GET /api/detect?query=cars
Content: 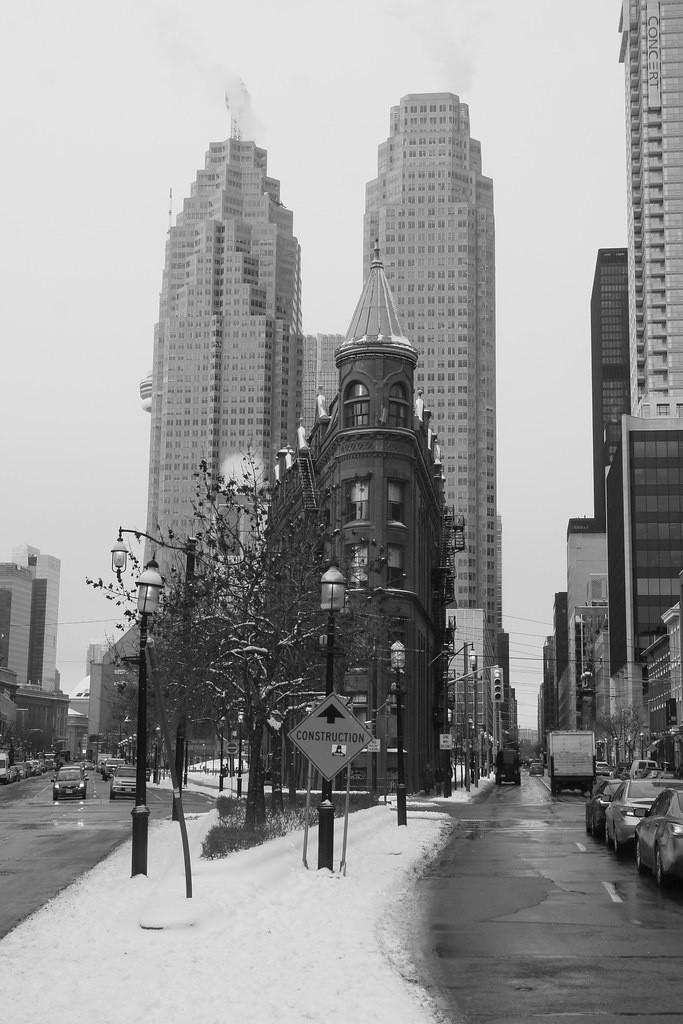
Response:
[51,761,89,800]
[604,779,683,855]
[585,779,632,839]
[110,764,148,800]
[97,757,127,781]
[635,787,683,889]
[82,761,95,771]
[595,759,683,779]
[10,759,48,782]
[529,763,544,776]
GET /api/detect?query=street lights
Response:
[468,716,500,784]
[131,553,164,872]
[111,526,198,821]
[389,637,407,826]
[316,553,347,870]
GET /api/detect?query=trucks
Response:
[496,749,520,785]
[44,753,57,770]
[546,729,595,796]
[0,753,11,784]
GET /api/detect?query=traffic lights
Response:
[490,668,504,702]
